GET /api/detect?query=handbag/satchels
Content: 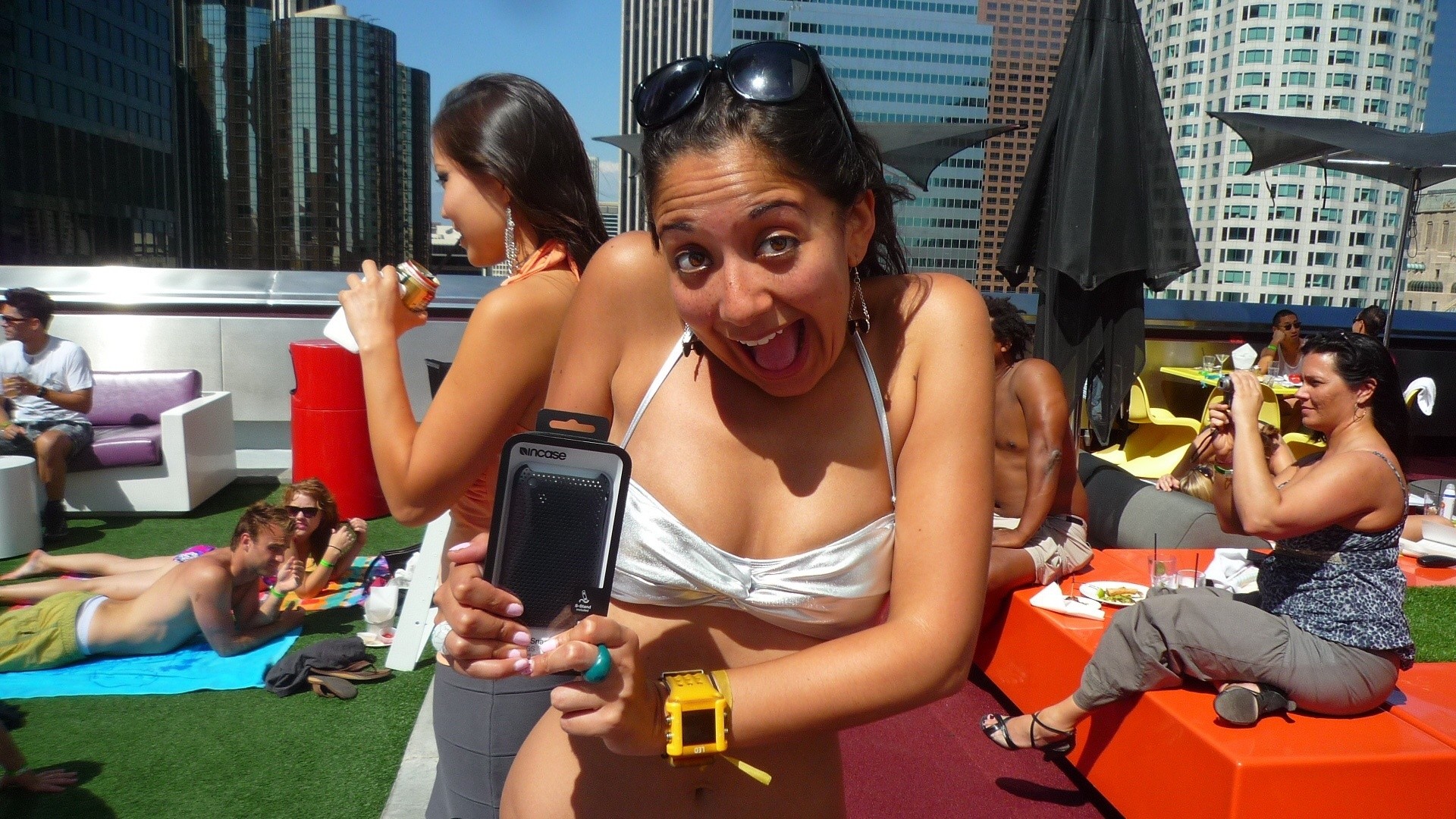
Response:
[364,541,423,583]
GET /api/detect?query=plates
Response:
[1079,581,1149,606]
[364,641,392,647]
[1063,595,1102,610]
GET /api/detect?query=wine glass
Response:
[1215,354,1229,377]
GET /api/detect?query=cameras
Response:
[1217,375,1234,408]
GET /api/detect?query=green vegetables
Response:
[1098,589,1136,603]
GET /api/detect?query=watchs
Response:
[658,669,772,786]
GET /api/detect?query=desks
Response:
[0,456,43,559]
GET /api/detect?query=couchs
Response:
[62,369,236,512]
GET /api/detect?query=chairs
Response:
[1081,366,1200,479]
[1201,371,1323,478]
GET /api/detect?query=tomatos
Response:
[1288,374,1302,384]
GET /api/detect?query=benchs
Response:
[999,544,1456,819]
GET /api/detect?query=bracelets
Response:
[36,386,47,398]
[319,559,336,567]
[1267,345,1277,351]
[1213,464,1233,474]
[270,587,287,598]
[0,421,13,430]
[327,545,342,553]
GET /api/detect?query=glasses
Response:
[1352,317,1362,324]
[1277,321,1302,330]
[1325,328,1357,358]
[284,505,319,519]
[0,313,30,326]
[633,39,852,163]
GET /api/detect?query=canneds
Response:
[395,258,440,314]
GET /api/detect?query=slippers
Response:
[310,658,391,680]
[307,676,358,700]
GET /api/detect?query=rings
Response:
[431,620,453,656]
[580,643,612,684]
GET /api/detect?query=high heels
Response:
[980,712,1076,761]
[1213,681,1298,725]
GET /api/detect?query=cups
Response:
[2,372,19,398]
[1253,366,1261,378]
[1203,356,1215,375]
[1148,554,1178,589]
[1424,493,1442,516]
[1262,374,1275,388]
[1057,573,1074,599]
[1177,569,1206,588]
[1268,361,1279,378]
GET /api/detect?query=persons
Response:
[336,71,610,819]
[1352,305,1397,364]
[429,42,996,819]
[0,477,368,673]
[1257,309,1309,375]
[979,328,1417,763]
[981,296,1094,635]
[1156,419,1298,504]
[0,287,97,536]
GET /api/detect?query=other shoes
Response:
[42,500,68,536]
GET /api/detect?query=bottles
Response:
[1439,483,1456,520]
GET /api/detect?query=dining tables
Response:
[1160,367,1302,395]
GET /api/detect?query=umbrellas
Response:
[1203,111,1456,351]
[591,123,1030,194]
[996,0,1202,473]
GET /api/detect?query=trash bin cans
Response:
[291,337,393,524]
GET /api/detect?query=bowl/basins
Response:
[357,632,377,644]
[1288,374,1302,383]
[379,627,397,643]
[1235,368,1251,372]
[366,614,394,637]
